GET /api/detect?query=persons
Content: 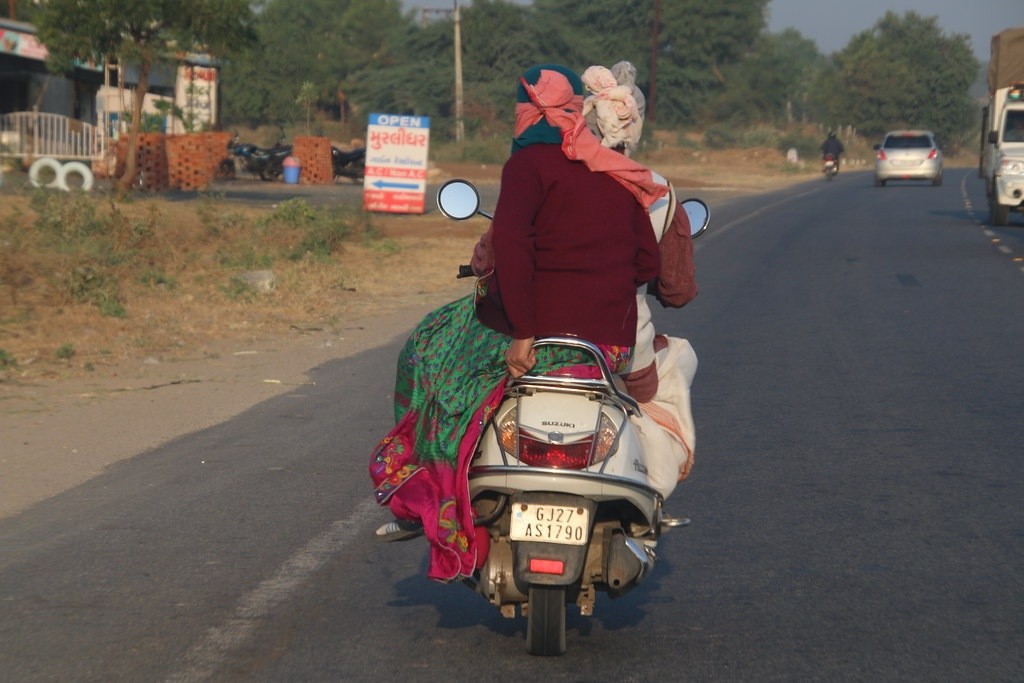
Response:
[369,64,659,587]
[473,62,697,496]
[822,131,844,170]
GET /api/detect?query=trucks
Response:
[978,82,1024,226]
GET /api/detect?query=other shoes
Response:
[375,520,418,541]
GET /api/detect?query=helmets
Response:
[829,131,836,138]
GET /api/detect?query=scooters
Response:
[822,153,839,182]
[437,178,711,656]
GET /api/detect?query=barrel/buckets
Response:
[283,165,301,185]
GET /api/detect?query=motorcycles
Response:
[214,130,293,182]
[331,146,365,181]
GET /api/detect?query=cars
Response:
[874,130,943,187]
